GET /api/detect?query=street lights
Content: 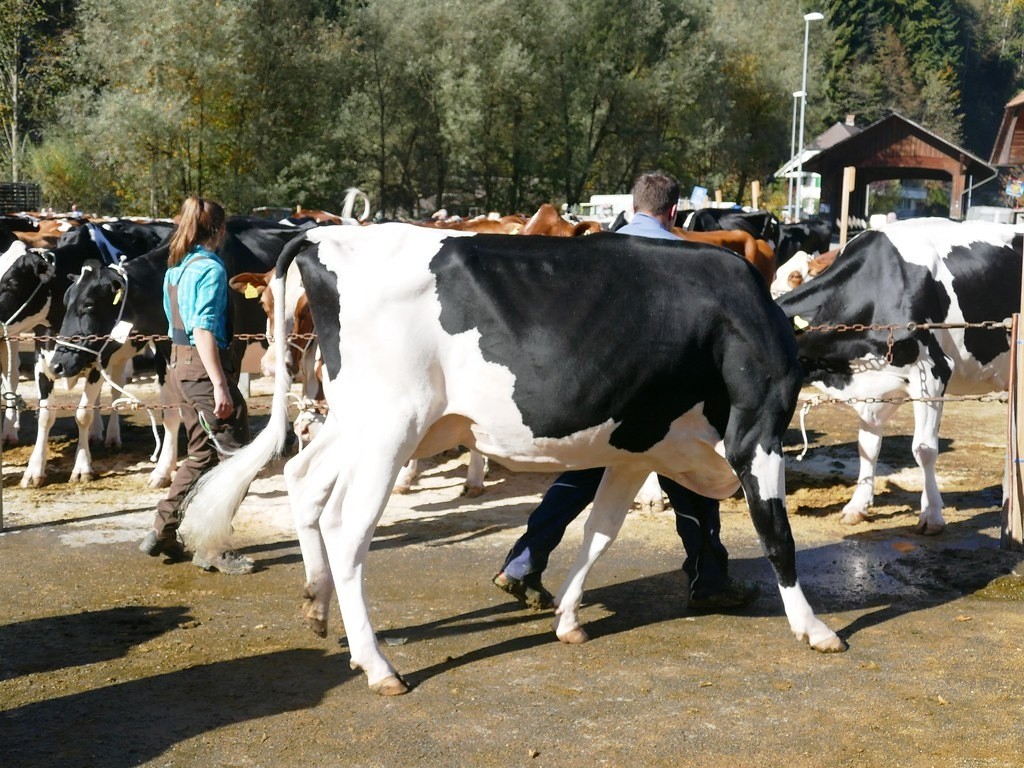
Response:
[785,91,807,225]
[794,12,826,221]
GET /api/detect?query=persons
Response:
[491,171,762,610]
[137,200,256,576]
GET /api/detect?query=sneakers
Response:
[493,570,552,608]
[193,547,255,575]
[689,577,760,609]
[139,530,184,558]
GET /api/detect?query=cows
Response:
[774,215,1023,535]
[0,184,862,489]
[178,220,849,697]
